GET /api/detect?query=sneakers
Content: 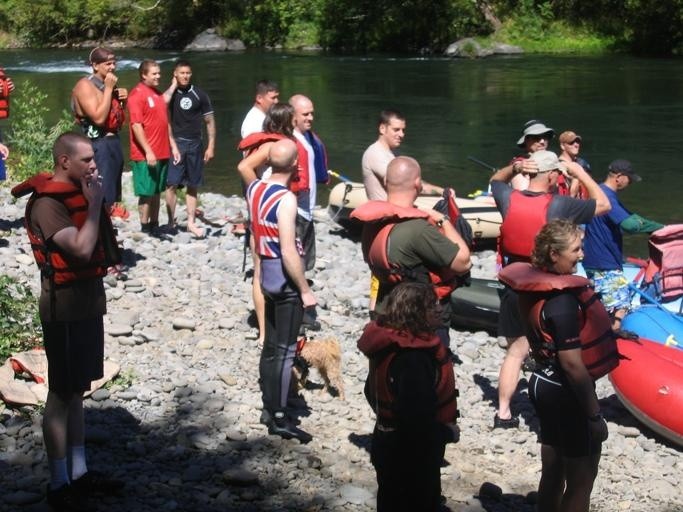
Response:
[260,410,301,427]
[268,421,313,443]
[495,415,519,428]
[302,314,321,331]
[46,483,98,510]
[76,474,124,496]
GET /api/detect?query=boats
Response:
[325,178,503,244]
[608,300,682,445]
[570,254,682,314]
[449,273,511,334]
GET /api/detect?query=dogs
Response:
[292,334,345,402]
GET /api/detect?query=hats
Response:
[517,118,555,144]
[559,131,583,146]
[608,158,642,183]
[84,46,115,64]
[530,151,563,172]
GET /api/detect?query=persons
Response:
[11,132,128,510]
[556,125,594,201]
[359,108,457,313]
[355,283,462,511]
[500,117,570,197]
[70,43,128,279]
[0,135,11,186]
[498,220,620,512]
[0,66,15,120]
[286,91,328,282]
[127,60,182,237]
[347,154,473,358]
[245,137,314,442]
[160,64,216,239]
[490,149,611,427]
[241,82,278,140]
[238,101,321,331]
[580,159,667,331]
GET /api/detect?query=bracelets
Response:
[586,412,604,423]
[512,164,521,174]
[436,215,452,228]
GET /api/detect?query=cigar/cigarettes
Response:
[90,174,104,179]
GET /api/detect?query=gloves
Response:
[445,422,460,444]
[577,410,608,441]
